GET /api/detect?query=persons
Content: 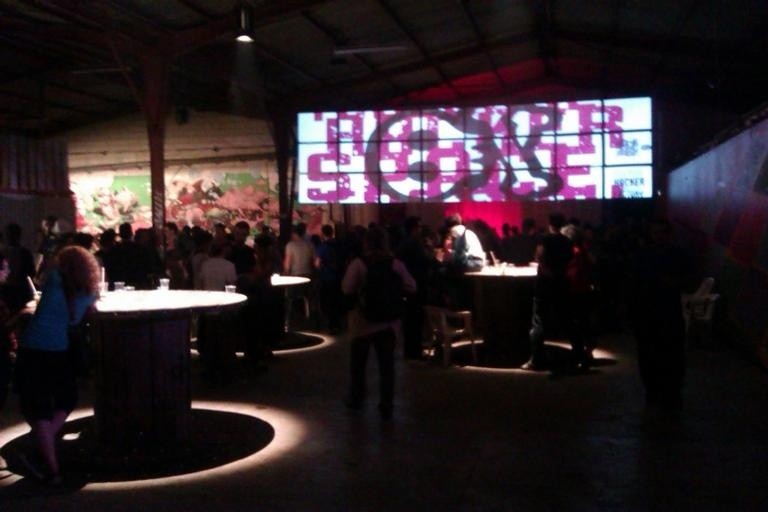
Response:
[0,208,723,428]
[11,243,109,495]
[624,217,720,444]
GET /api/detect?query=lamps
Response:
[232,1,257,44]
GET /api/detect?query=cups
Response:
[224,285,236,297]
[159,277,170,293]
[113,281,126,292]
[126,287,136,293]
[97,282,110,299]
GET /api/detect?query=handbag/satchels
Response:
[66,318,108,372]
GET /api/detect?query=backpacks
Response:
[355,255,405,322]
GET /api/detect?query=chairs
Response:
[423,303,479,366]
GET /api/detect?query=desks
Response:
[453,262,539,368]
[88,288,249,431]
[269,275,312,347]
